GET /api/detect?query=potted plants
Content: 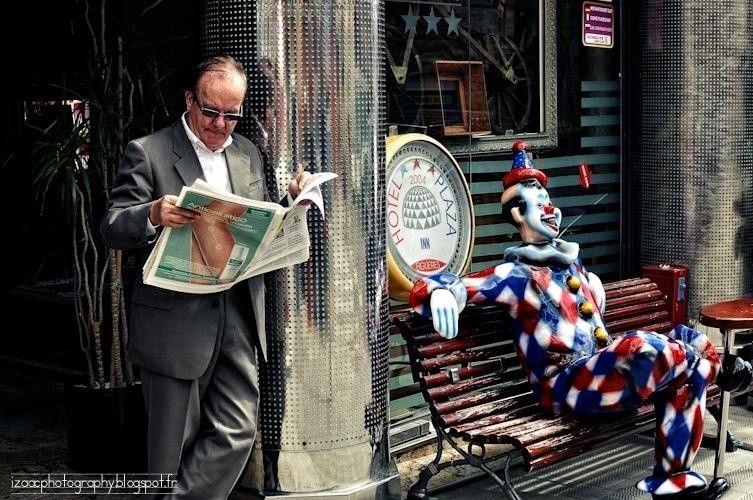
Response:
[20,0,172,473]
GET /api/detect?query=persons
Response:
[99,58,319,499]
[410,138,753,500]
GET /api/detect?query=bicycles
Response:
[384,5,532,134]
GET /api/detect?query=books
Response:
[142,171,338,296]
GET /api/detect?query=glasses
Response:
[193,94,244,122]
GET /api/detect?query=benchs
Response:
[387,264,738,500]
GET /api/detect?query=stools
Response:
[698,298,753,494]
